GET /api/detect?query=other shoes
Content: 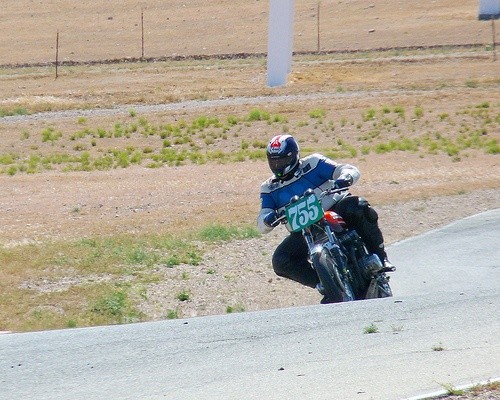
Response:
[316,283,326,295]
[383,259,396,271]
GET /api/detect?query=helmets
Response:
[266,134,299,177]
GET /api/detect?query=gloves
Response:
[264,210,280,227]
[334,173,353,195]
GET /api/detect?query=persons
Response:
[256,133,398,303]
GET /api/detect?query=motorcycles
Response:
[270,180,396,303]
